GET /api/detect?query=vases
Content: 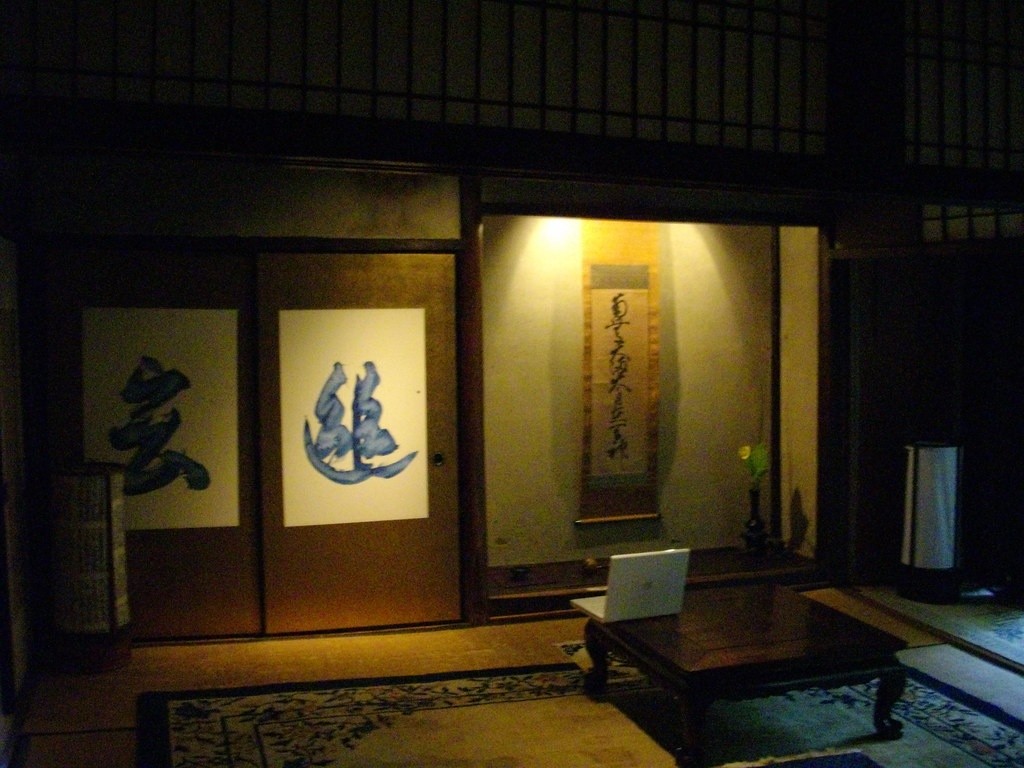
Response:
[740,488,768,551]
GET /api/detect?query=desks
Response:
[584,583,910,768]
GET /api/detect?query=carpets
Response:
[552,639,648,691]
[136,661,1024,768]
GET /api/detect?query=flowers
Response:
[739,443,770,489]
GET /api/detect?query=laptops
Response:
[570,548,690,624]
